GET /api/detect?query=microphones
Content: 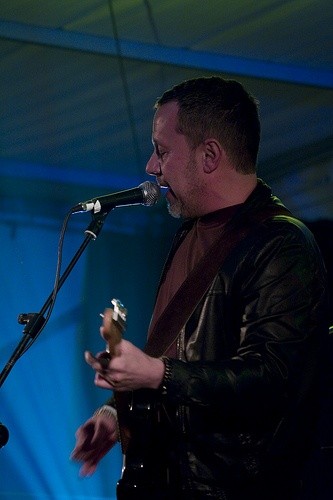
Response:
[71,181,162,214]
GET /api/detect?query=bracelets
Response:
[99,404,122,446]
[158,356,173,396]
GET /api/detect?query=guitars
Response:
[98,299,176,500]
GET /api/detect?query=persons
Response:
[73,77,333,500]
